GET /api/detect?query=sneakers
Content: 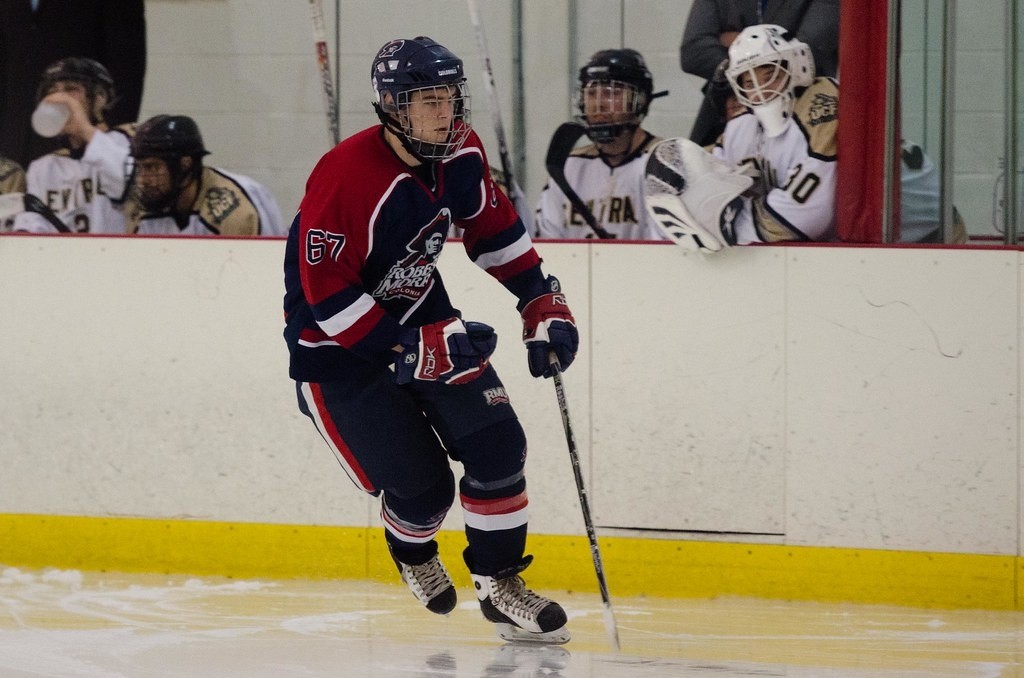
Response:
[385,529,458,617]
[462,546,570,645]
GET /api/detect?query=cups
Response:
[31,96,71,137]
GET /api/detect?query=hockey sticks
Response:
[544,121,618,243]
[548,350,620,650]
[0,191,71,233]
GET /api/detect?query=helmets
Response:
[725,23,816,139]
[571,48,671,143]
[123,114,210,209]
[369,37,472,164]
[37,57,115,107]
[709,59,736,111]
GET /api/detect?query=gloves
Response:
[516,274,578,378]
[394,316,498,386]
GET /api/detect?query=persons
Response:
[283,35,579,644]
[446,48,668,241]
[15,56,140,234]
[645,0,965,248]
[0,154,26,232]
[124,113,288,236]
